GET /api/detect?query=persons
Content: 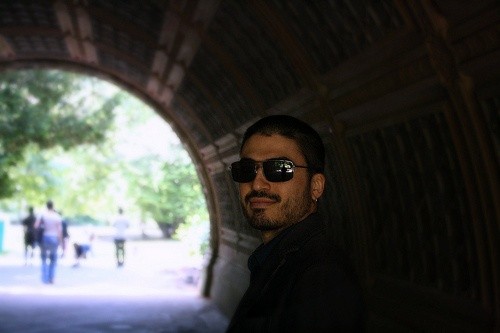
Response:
[21,200,94,284]
[225,114,368,333]
[112,207,130,267]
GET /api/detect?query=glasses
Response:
[228,159,307,183]
